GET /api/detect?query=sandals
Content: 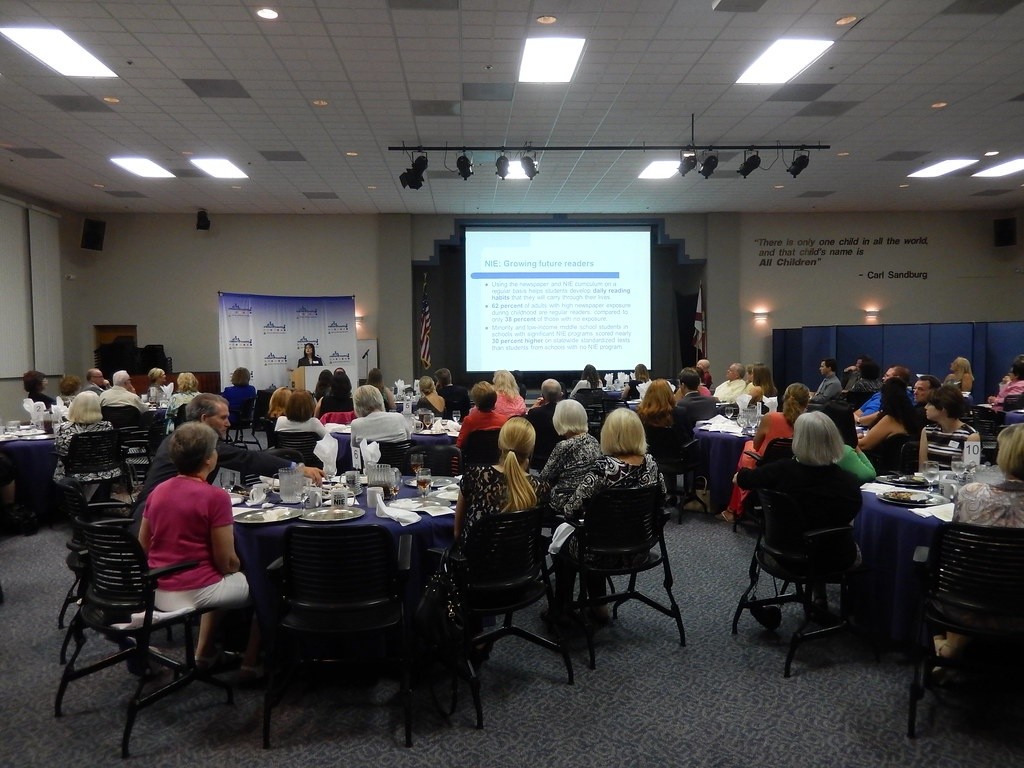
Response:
[194,647,222,672]
[238,664,265,682]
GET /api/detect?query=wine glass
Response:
[416,468,432,503]
[411,454,423,484]
[922,461,939,493]
[951,461,966,484]
[725,405,763,436]
[322,466,337,486]
[423,414,433,429]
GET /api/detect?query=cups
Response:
[220,471,235,493]
[404,408,461,434]
[939,462,1005,500]
[0,412,62,436]
[367,487,384,508]
[250,483,269,504]
[140,387,170,409]
[393,392,422,403]
[340,471,360,494]
[303,487,322,508]
[331,489,355,510]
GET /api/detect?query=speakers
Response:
[994,218,1017,247]
[81,220,106,251]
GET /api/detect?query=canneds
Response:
[856,427,864,435]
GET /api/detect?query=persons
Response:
[298,343,323,368]
[569,363,603,398]
[221,367,256,424]
[636,359,712,509]
[268,388,292,423]
[528,379,566,472]
[427,399,666,640]
[712,356,1024,696]
[350,384,411,447]
[165,372,202,434]
[23,370,68,413]
[0,453,15,503]
[417,368,527,477]
[60,374,81,408]
[274,389,327,440]
[126,392,328,518]
[138,421,263,673]
[99,370,149,414]
[621,364,649,400]
[52,390,122,502]
[367,368,397,412]
[314,367,354,419]
[148,367,170,404]
[82,368,112,395]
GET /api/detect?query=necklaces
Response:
[190,475,205,483]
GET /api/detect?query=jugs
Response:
[272,466,304,502]
[366,464,400,499]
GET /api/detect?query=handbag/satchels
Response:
[414,549,464,645]
[683,476,710,513]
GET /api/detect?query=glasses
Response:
[211,445,220,453]
[90,376,102,378]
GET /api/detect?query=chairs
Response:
[50,387,1024,758]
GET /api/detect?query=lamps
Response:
[678,150,697,177]
[399,151,429,189]
[196,210,210,231]
[699,149,720,179]
[736,149,762,180]
[496,150,513,180]
[786,146,812,178]
[457,151,475,180]
[520,151,539,180]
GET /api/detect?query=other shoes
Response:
[715,509,735,523]
[581,604,610,624]
[813,597,829,610]
[540,606,581,630]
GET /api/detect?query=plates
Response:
[413,429,448,434]
[875,472,951,505]
[395,400,403,404]
[232,474,460,524]
[0,425,59,441]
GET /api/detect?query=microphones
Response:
[362,350,369,359]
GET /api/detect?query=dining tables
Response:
[220,474,459,637]
[691,419,760,504]
[1,438,61,517]
[326,426,460,478]
[978,403,1024,424]
[848,469,970,647]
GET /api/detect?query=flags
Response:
[695,286,707,359]
[420,282,431,370]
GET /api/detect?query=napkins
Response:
[604,372,636,386]
[374,492,422,527]
[23,394,68,422]
[312,433,339,475]
[445,420,462,432]
[430,419,444,432]
[395,379,405,395]
[358,439,381,471]
[160,382,174,396]
